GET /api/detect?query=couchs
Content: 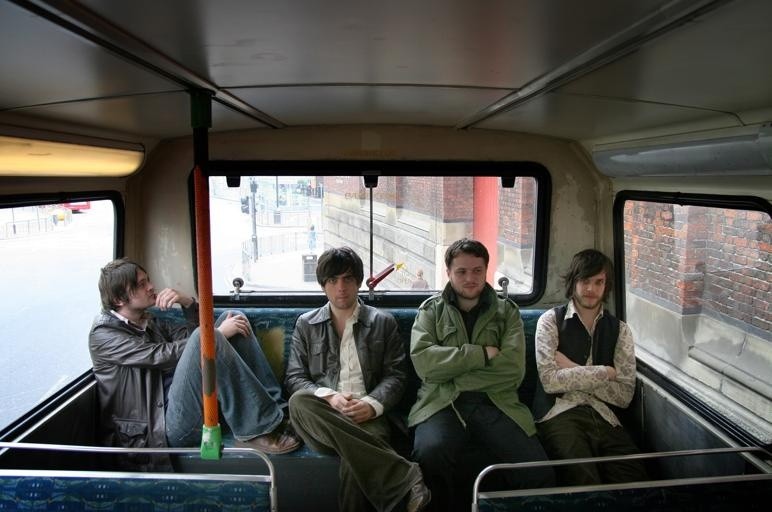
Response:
[240,196,249,214]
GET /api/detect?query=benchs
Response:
[1,441,276,509]
[148,306,675,509]
[472,447,772,510]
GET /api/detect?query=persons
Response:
[406,237,557,512]
[532,247,648,493]
[87,258,305,473]
[306,225,318,254]
[411,269,429,289]
[282,245,432,512]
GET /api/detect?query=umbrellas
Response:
[402,480,432,512]
[234,430,304,455]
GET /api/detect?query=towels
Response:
[301,253,318,283]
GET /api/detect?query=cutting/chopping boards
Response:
[412,269,429,291]
